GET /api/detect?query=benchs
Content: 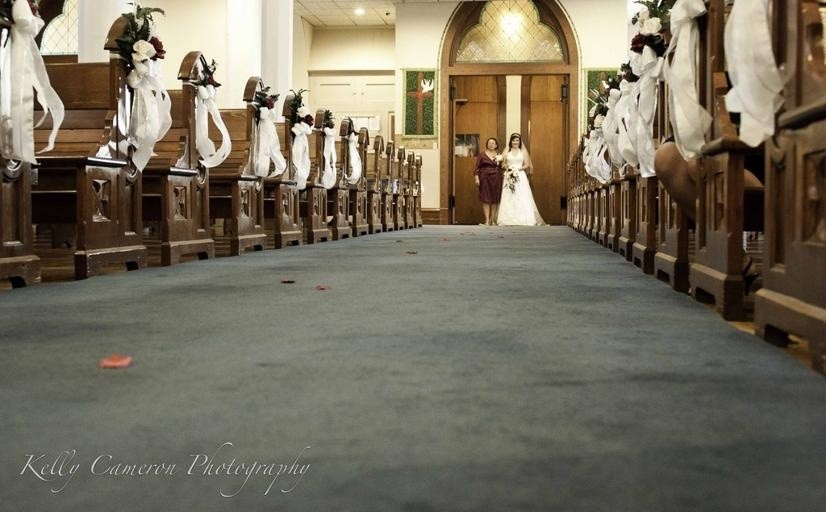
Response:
[566,0,826,374]
[0,0,424,294]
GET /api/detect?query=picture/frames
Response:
[402,67,439,139]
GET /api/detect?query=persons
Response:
[495,132,535,226]
[654,134,764,294]
[473,136,504,226]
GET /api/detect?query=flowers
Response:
[503,167,519,193]
